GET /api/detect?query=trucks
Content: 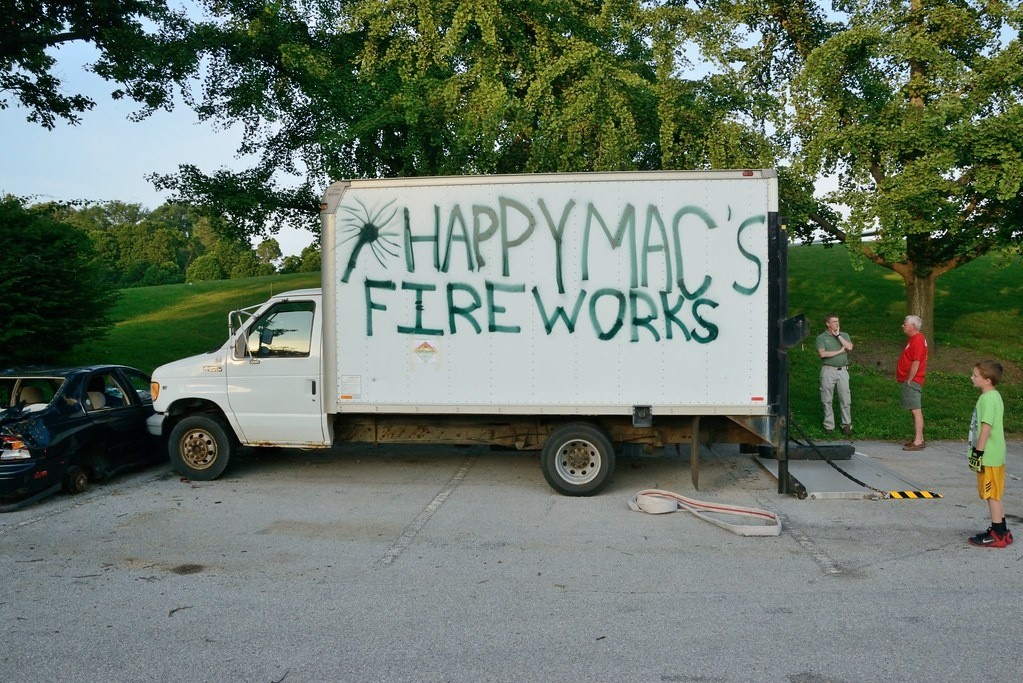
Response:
[145,167,805,498]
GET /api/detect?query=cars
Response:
[0,364,163,503]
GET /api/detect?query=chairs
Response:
[87,392,106,409]
[20,386,41,407]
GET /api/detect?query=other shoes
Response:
[843,425,851,435]
[903,443,923,451]
[904,439,926,448]
[826,429,832,434]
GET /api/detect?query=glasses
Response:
[828,320,839,323]
[903,321,911,325]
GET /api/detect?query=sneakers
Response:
[975,526,1014,544]
[968,526,1007,548]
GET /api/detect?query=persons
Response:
[896,315,928,451]
[815,312,853,435]
[967,360,1014,547]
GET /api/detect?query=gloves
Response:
[969,447,984,473]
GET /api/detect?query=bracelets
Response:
[836,333,841,338]
